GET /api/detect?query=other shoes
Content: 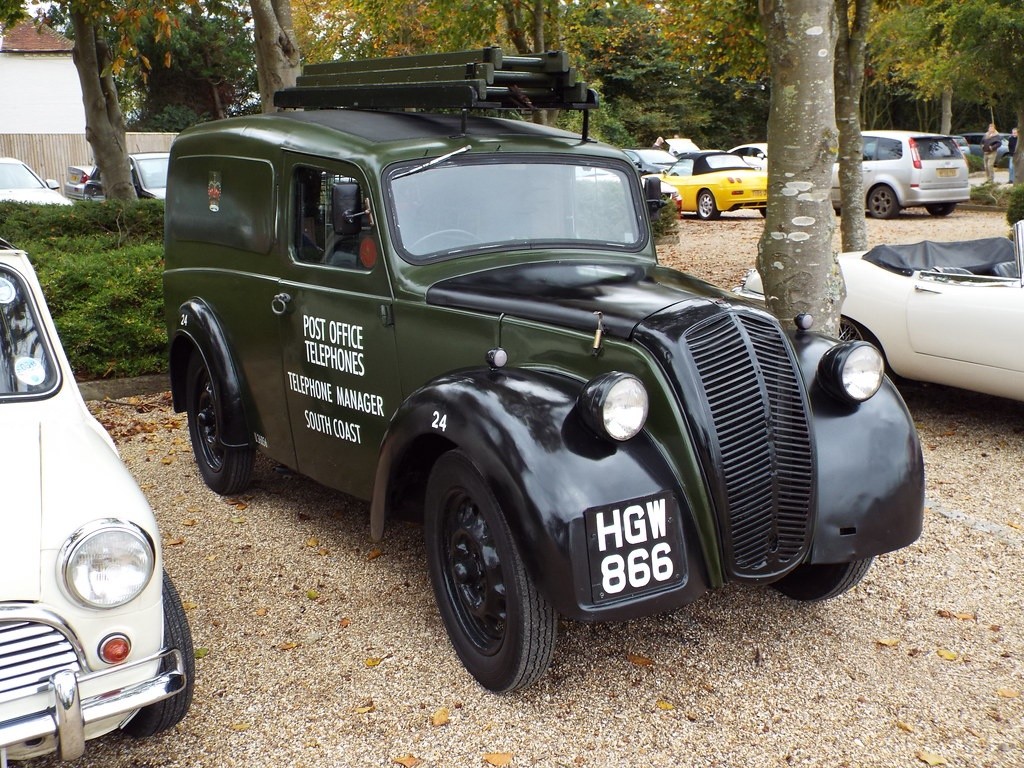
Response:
[1007,181,1013,184]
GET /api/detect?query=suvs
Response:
[831,127,971,220]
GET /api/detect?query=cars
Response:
[0,240,196,767]
[619,147,679,177]
[64,164,95,201]
[82,151,171,202]
[164,42,925,696]
[575,168,682,226]
[1,157,77,206]
[951,135,971,156]
[727,142,768,173]
[962,133,1014,167]
[639,150,769,220]
[730,219,1024,401]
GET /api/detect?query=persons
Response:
[653,136,664,147]
[981,124,1002,185]
[1007,128,1019,183]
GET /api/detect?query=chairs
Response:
[992,260,1018,278]
[931,266,974,275]
